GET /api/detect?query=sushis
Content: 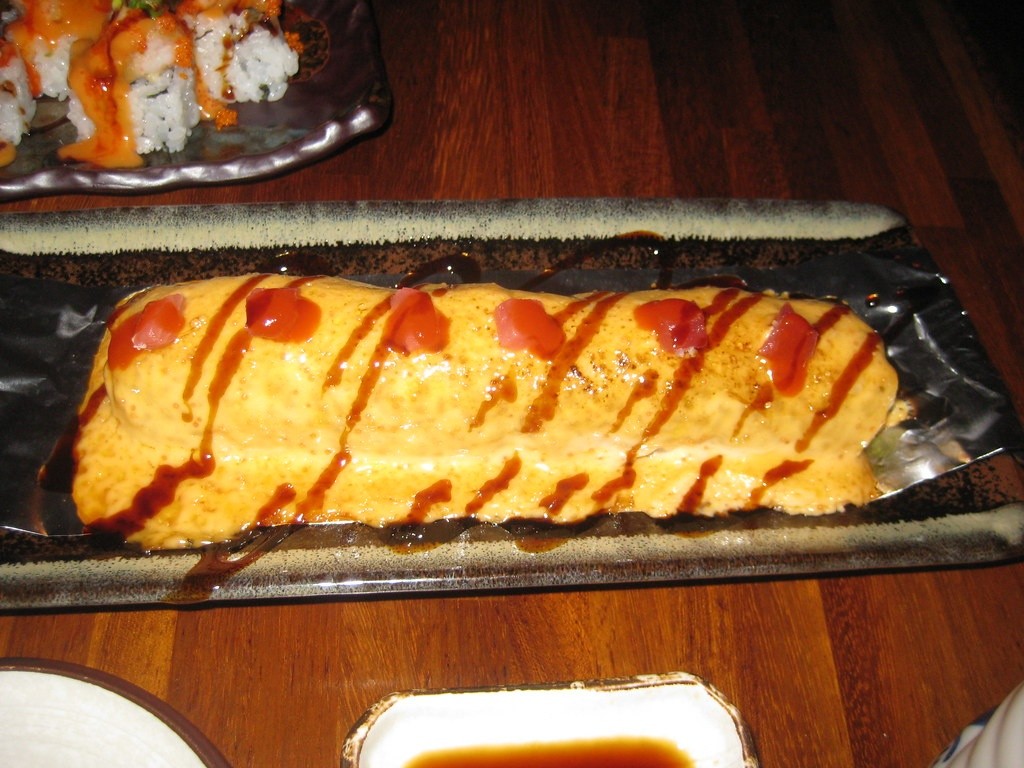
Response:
[0,0,309,170]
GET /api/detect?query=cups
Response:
[926,677,1023,766]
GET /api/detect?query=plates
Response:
[0,198,1022,609]
[0,657,231,767]
[338,669,761,768]
[1,1,399,200]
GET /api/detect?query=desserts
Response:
[37,273,896,553]
